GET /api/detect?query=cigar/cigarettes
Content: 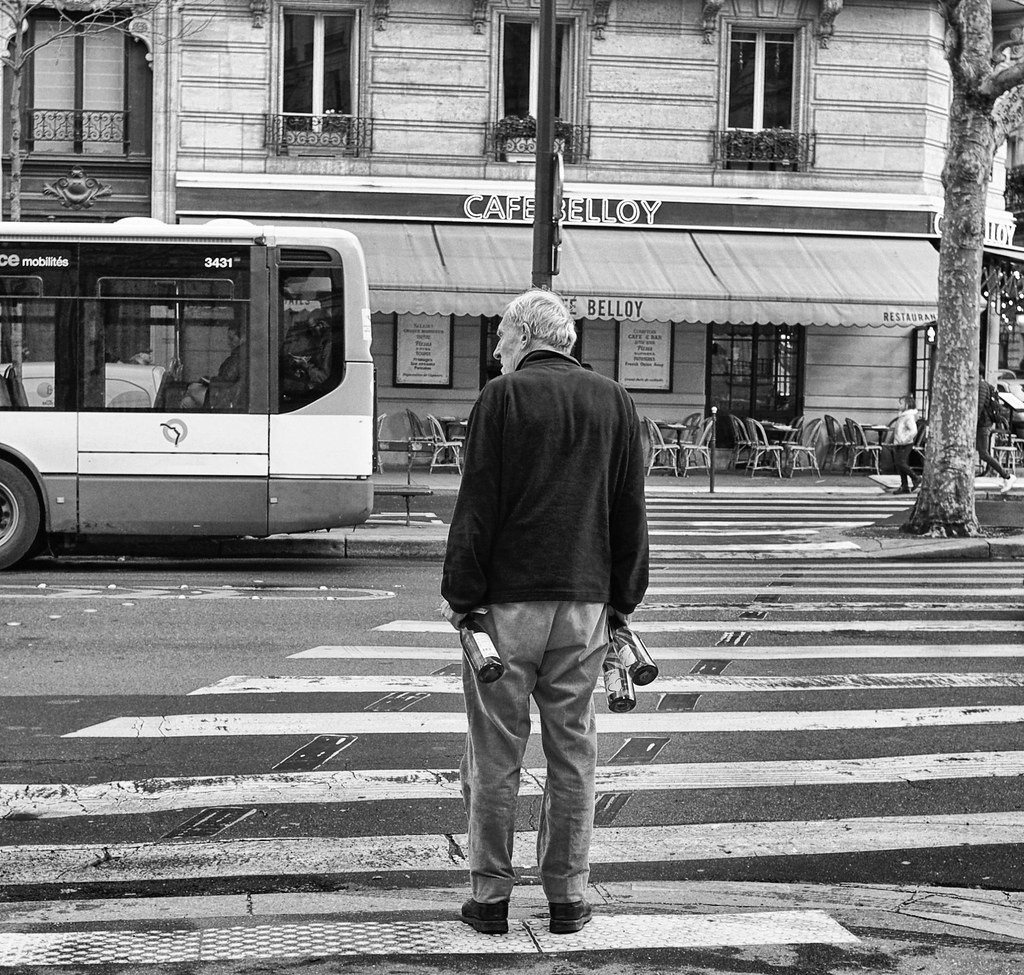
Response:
[434,609,443,612]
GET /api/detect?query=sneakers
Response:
[1001,474,1016,492]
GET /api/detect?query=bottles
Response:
[459,614,506,684]
[606,615,658,685]
[601,643,637,714]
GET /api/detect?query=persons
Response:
[891,395,922,494]
[280,302,319,379]
[181,319,246,411]
[130,339,154,368]
[290,310,337,389]
[974,363,1017,495]
[436,283,651,936]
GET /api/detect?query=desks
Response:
[656,423,701,478]
[438,418,469,464]
[763,423,799,477]
[843,422,890,475]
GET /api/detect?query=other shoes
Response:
[894,485,909,494]
[911,476,921,492]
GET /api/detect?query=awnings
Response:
[176,200,990,332]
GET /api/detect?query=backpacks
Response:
[984,382,1000,422]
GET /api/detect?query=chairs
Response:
[744,417,785,479]
[782,417,824,480]
[643,415,681,478]
[842,417,882,476]
[670,415,718,478]
[768,416,806,478]
[818,414,859,475]
[406,408,441,472]
[426,412,464,475]
[727,415,765,474]
[661,412,702,476]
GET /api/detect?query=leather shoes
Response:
[463,898,511,932]
[550,897,591,934]
